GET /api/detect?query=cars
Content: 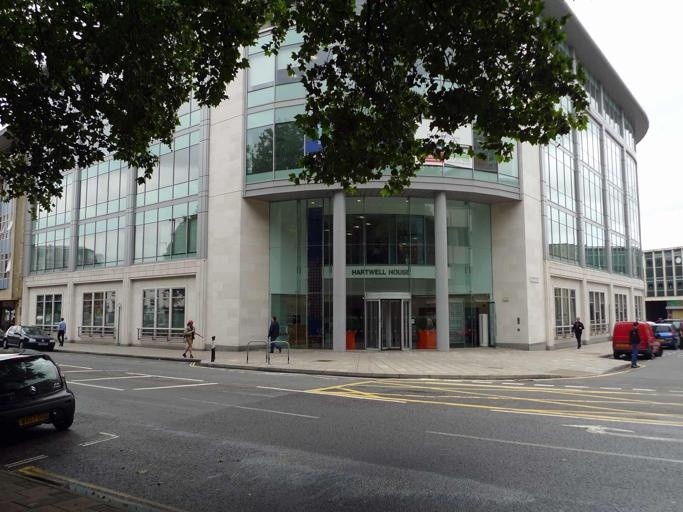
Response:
[612,318,683,358]
[3,323,55,352]
[0,328,5,346]
[0,351,77,439]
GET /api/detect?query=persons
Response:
[57,318,66,346]
[268,317,281,353]
[630,322,640,368]
[572,318,584,349]
[183,320,195,358]
